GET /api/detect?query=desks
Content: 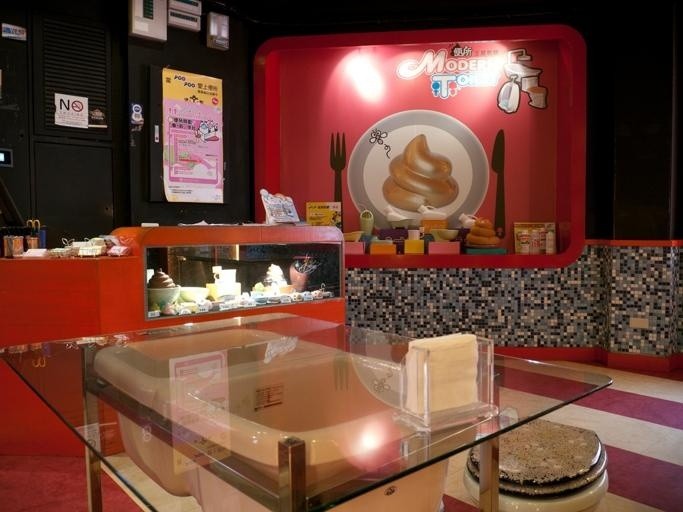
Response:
[1,311,612,512]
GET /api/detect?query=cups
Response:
[214,269,237,284]
[408,230,425,240]
[280,285,294,304]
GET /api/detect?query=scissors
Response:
[26,220,40,234]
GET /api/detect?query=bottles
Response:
[519,228,556,255]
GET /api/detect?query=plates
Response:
[347,109,490,231]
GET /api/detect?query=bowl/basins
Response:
[256,297,280,305]
[437,229,459,241]
[147,284,209,305]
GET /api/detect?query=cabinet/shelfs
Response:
[0,0,117,251]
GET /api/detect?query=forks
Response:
[329,132,346,233]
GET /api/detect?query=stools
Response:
[463,416,609,512]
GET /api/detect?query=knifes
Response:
[491,127,506,238]
